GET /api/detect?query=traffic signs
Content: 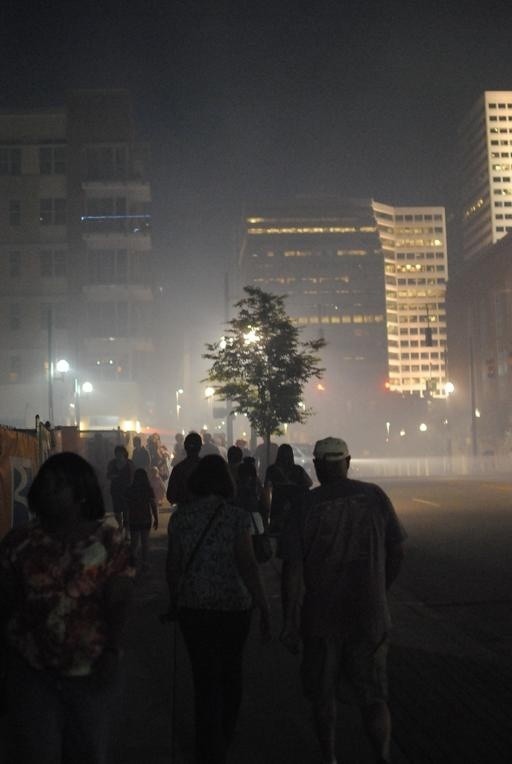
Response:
[487,357,495,377]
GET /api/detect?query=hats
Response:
[312,436,350,463]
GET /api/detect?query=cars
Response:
[285,441,320,481]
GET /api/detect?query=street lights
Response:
[48,357,70,427]
[434,381,457,458]
[204,383,235,450]
[74,379,98,426]
[175,387,186,432]
